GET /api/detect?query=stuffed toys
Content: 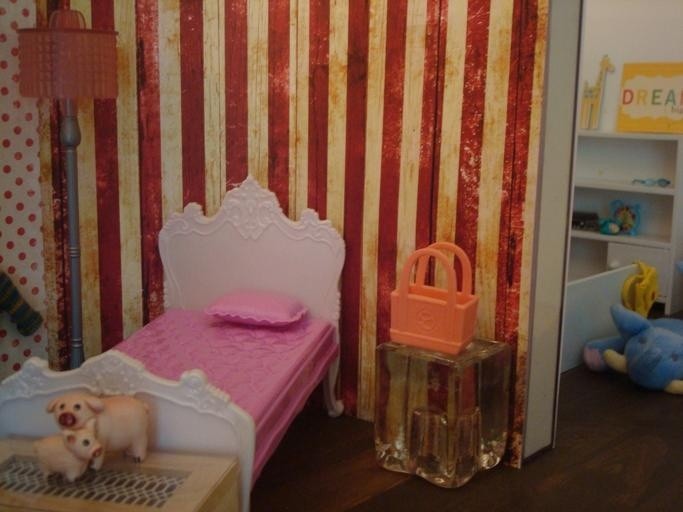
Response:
[598,197,642,237]
[583,302,682,393]
[46,393,151,473]
[33,417,103,484]
